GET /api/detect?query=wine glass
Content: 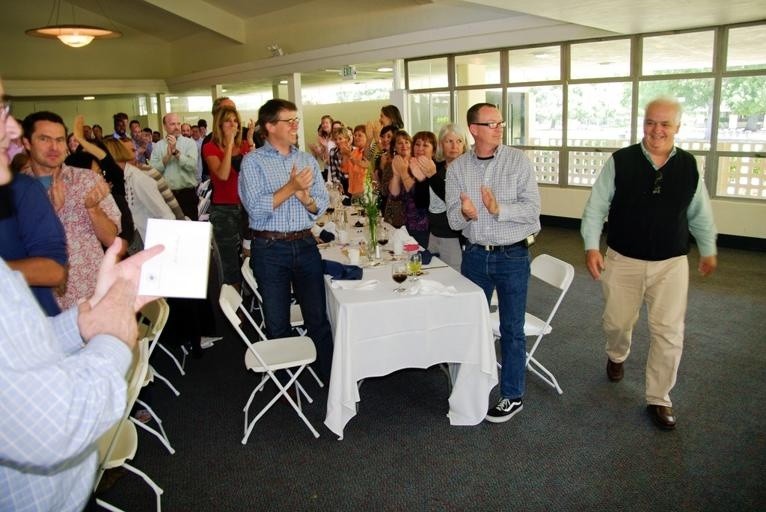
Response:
[319,179,426,297]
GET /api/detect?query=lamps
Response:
[24,0,123,48]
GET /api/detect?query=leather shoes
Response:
[647,405,676,432]
[606,357,625,383]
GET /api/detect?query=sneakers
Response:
[134,409,153,423]
[272,382,299,407]
[485,397,523,424]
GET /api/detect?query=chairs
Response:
[490,253,575,396]
[216,254,325,446]
[93,298,191,512]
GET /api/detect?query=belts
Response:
[473,241,526,251]
[252,228,312,242]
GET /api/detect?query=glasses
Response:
[269,116,300,124]
[647,170,664,199]
[0,92,13,121]
[470,120,507,129]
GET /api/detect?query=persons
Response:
[12,97,467,307]
[21,109,154,424]
[0,140,68,316]
[238,98,333,393]
[579,95,719,431]
[445,103,542,425]
[0,85,166,512]
